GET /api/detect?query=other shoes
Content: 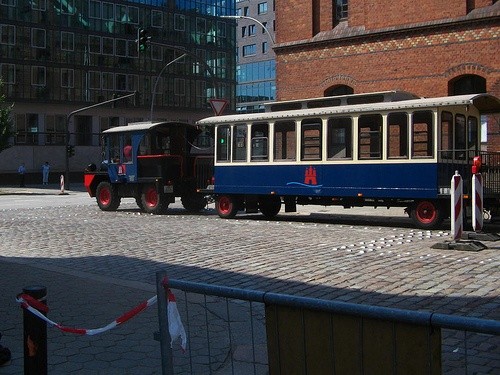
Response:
[46,183,48,185]
[43,183,45,185]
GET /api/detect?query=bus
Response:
[84,90,500,219]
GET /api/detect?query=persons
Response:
[18,161,50,188]
